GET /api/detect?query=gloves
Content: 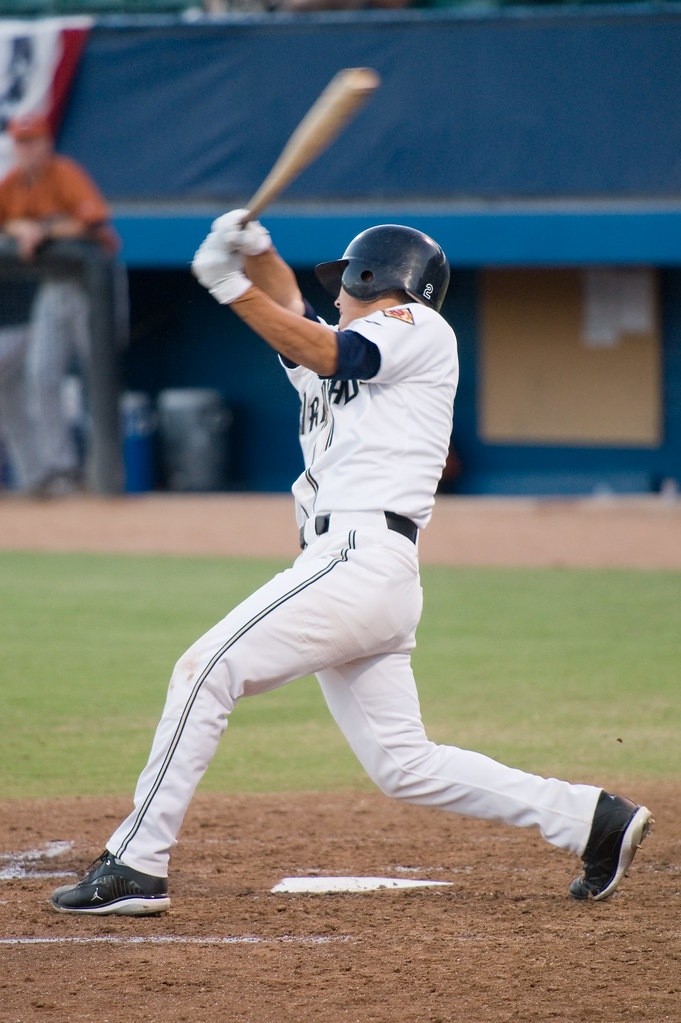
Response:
[192,208,271,305]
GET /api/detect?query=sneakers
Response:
[51,850,171,916]
[568,790,656,901]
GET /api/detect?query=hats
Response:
[10,110,54,143]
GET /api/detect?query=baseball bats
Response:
[235,61,381,227]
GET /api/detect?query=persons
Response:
[0,112,108,495]
[51,209,653,915]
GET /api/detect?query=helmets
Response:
[314,223,450,313]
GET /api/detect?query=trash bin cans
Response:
[83,392,157,494]
[161,387,233,491]
